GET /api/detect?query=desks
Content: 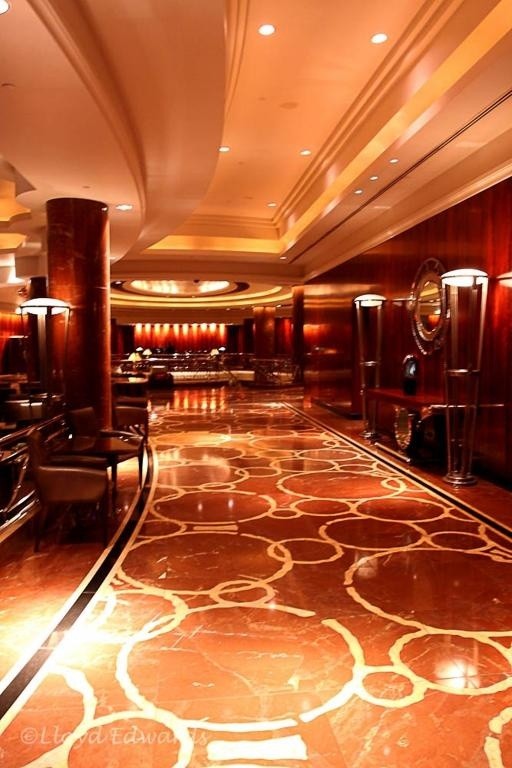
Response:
[366,386,445,448]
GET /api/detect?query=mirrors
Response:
[408,258,450,358]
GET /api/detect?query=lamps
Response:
[352,294,388,440]
[18,298,71,406]
[439,268,489,487]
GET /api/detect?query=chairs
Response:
[30,405,150,552]
